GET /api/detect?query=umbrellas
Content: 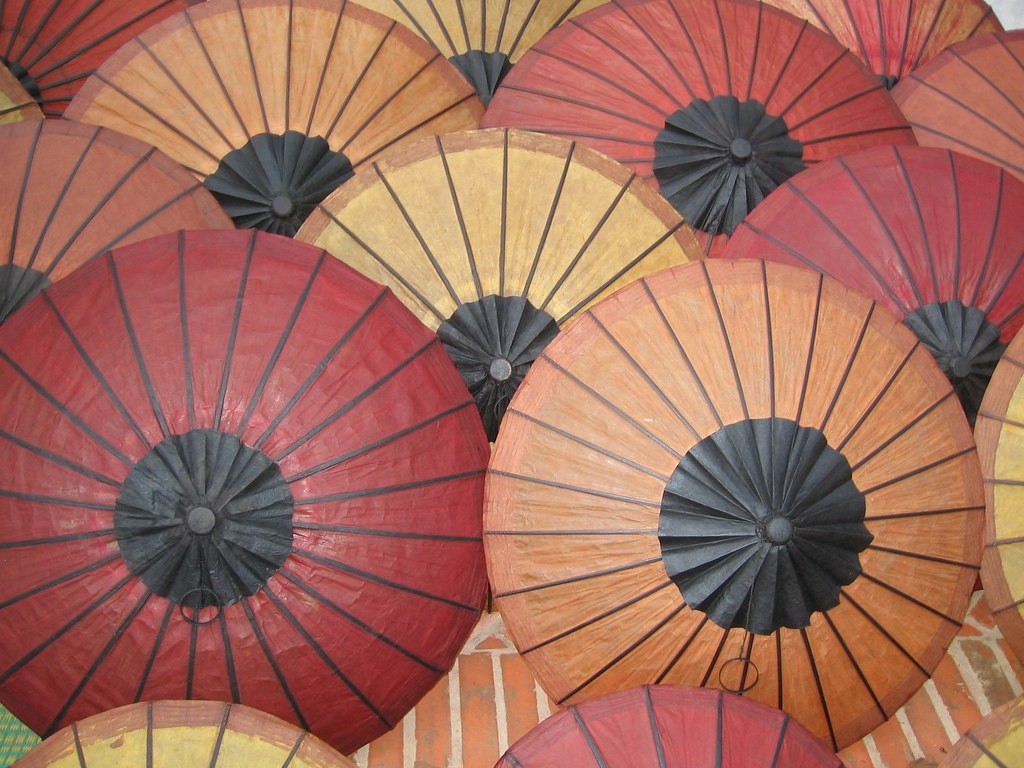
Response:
[482,256,985,753]
[0,228,491,756]
[0,0,1024,768]
